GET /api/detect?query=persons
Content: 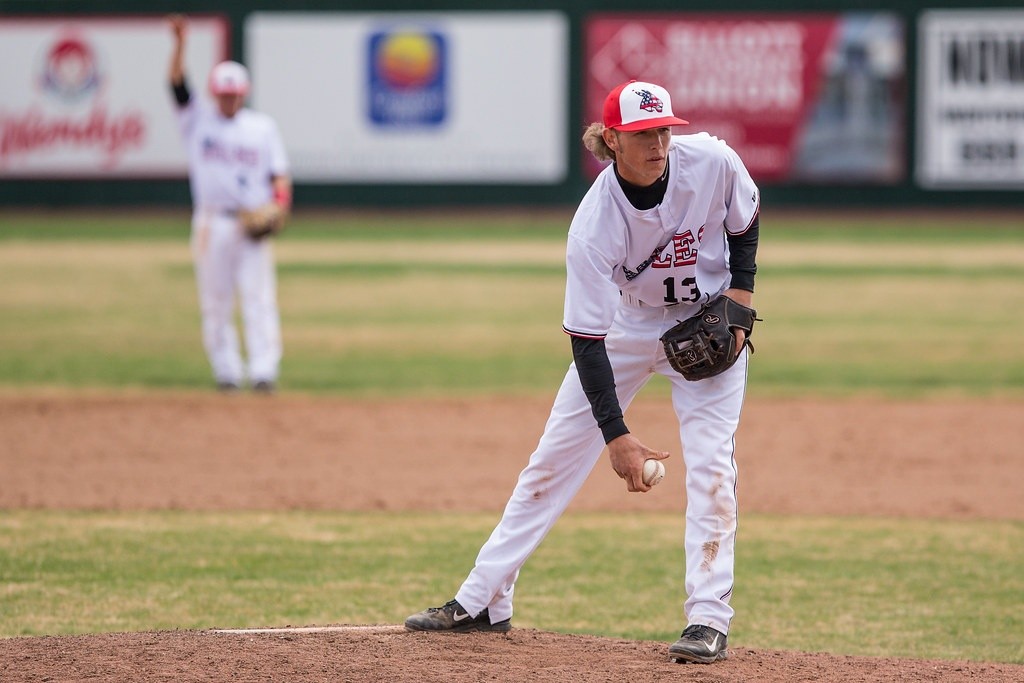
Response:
[403,79,761,665]
[167,13,293,391]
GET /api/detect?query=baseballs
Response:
[643,458,665,486]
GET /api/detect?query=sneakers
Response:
[668,625,728,664]
[405,599,512,633]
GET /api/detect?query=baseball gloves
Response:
[661,294,758,382]
[239,202,289,238]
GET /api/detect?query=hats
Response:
[210,61,249,95]
[603,80,689,132]
